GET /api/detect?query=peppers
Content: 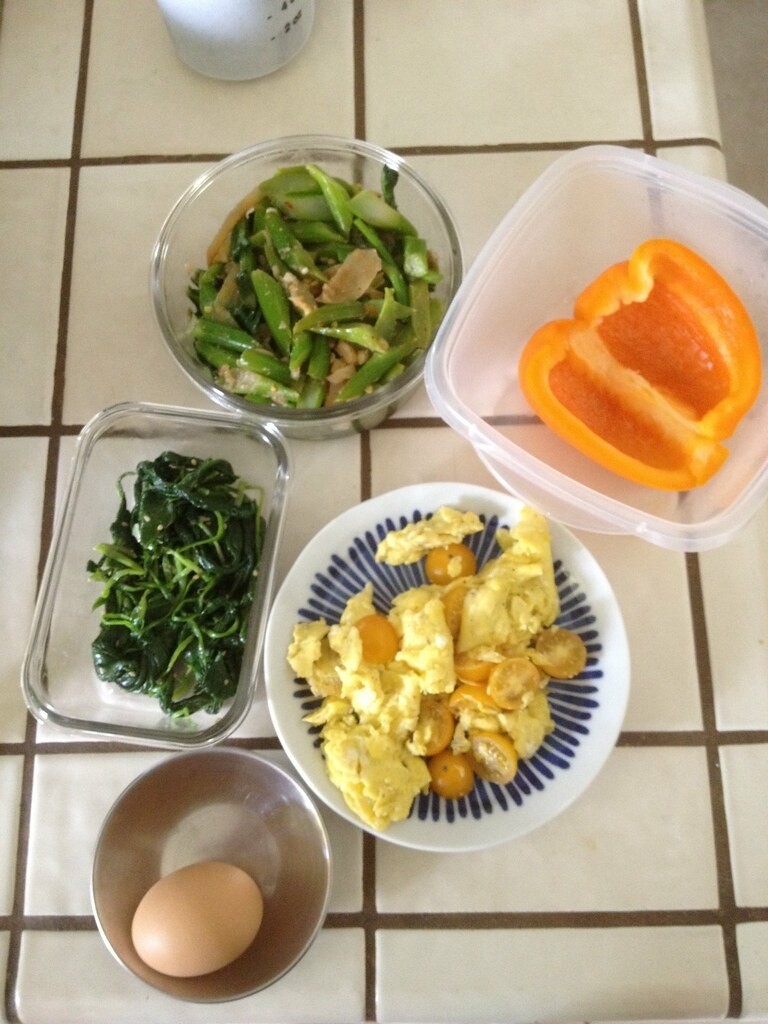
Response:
[517,235,763,493]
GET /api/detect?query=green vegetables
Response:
[84,450,266,720]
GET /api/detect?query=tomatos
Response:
[355,545,587,800]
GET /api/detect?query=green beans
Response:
[185,164,446,411]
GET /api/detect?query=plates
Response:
[259,478,630,853]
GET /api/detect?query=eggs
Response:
[131,860,264,979]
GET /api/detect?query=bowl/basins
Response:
[20,401,293,752]
[421,144,768,551]
[149,136,464,444]
[87,745,334,1008]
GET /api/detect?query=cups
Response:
[153,0,316,82]
